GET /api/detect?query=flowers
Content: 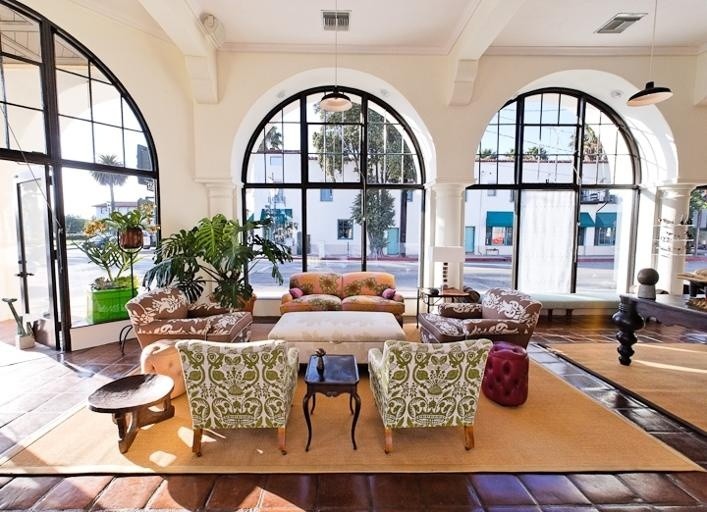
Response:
[84,199,161,237]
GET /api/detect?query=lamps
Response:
[428,245,464,289]
[313,0,360,118]
[623,2,677,111]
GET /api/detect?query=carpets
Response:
[533,333,707,441]
[0,361,706,477]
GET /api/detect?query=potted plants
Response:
[74,240,138,323]
[312,349,329,372]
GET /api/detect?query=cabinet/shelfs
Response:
[652,213,697,262]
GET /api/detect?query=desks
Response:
[88,370,179,454]
[299,348,367,456]
[417,284,475,315]
[614,292,707,366]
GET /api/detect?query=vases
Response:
[118,229,144,248]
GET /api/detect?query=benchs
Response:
[527,293,618,321]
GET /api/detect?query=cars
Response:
[78,234,111,250]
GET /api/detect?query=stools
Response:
[481,339,534,408]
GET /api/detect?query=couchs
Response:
[416,286,546,357]
[123,286,256,350]
[170,335,304,459]
[276,269,407,330]
[365,336,496,454]
[264,309,408,368]
